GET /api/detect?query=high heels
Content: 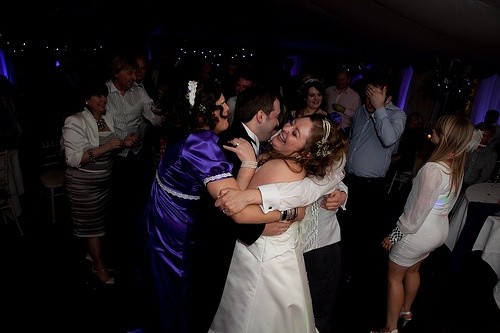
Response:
[90,265,115,285]
[399,311,412,326]
[85,253,115,271]
[370,328,398,333]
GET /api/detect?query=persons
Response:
[463,122,496,190]
[146,58,352,333]
[484,110,500,124]
[387,112,422,173]
[333,76,406,178]
[325,67,360,134]
[62,81,141,284]
[370,114,476,333]
[104,53,165,159]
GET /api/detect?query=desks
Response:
[444,184,500,324]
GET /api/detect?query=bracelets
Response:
[241,161,258,168]
[88,148,96,163]
[279,208,298,221]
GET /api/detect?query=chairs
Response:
[0,149,25,237]
[31,143,69,224]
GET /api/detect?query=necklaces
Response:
[97,115,106,130]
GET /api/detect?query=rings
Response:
[223,210,226,213]
[236,141,240,147]
[384,241,386,243]
